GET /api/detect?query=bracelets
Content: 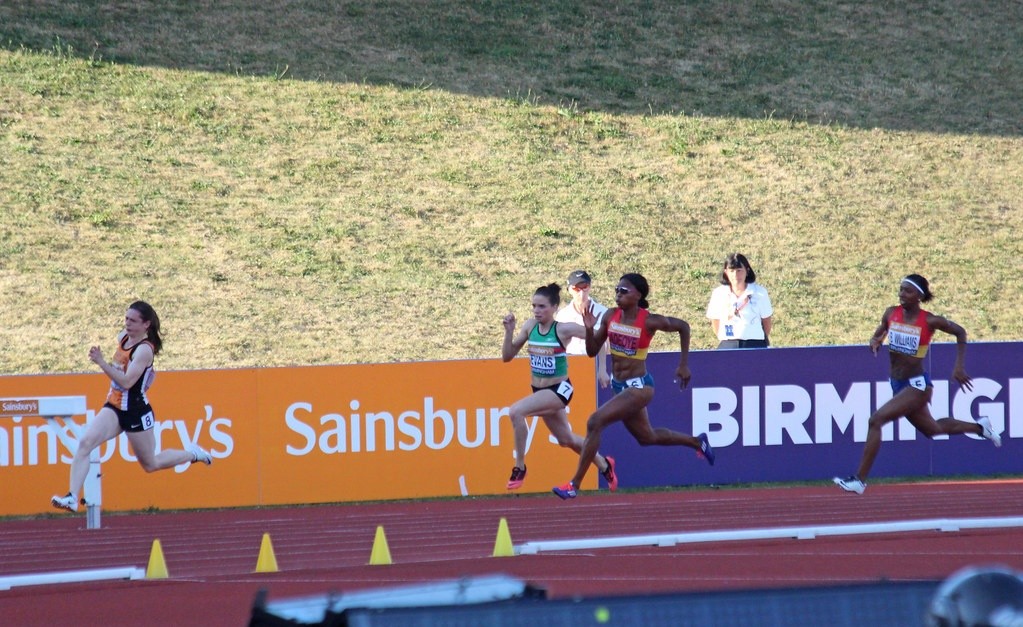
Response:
[957,342,968,345]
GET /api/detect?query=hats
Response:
[567,271,591,287]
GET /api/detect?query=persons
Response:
[554,270,609,354]
[832,274,1001,495]
[552,272,715,499]
[705,252,773,349]
[51,298,212,513]
[502,281,617,493]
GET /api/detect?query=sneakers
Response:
[507,464,527,489]
[976,416,1002,449]
[552,482,578,499]
[185,443,212,465]
[697,433,717,465]
[52,492,79,513]
[833,474,868,495]
[600,456,618,492]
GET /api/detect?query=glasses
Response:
[569,284,591,292]
[615,287,636,294]
